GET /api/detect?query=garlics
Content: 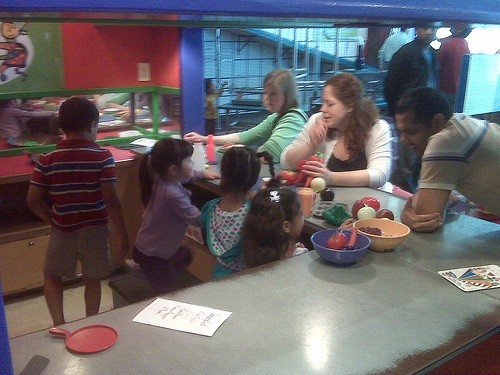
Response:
[357,203,376,219]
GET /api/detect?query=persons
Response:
[200,147,282,282]
[280,72,392,189]
[109,91,173,125]
[383,24,443,121]
[394,88,500,233]
[436,23,473,120]
[90,93,130,114]
[26,97,129,328]
[382,26,416,69]
[0,98,59,152]
[241,186,310,270]
[204,78,230,139]
[181,68,308,164]
[133,138,202,294]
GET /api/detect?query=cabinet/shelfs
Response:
[105,168,147,273]
[0,11,63,96]
[63,9,181,96]
[0,223,85,295]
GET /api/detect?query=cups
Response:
[296,188,314,217]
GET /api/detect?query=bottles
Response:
[192,139,204,172]
[205,134,215,162]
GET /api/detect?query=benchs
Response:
[219,99,269,131]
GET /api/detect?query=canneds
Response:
[296,188,314,215]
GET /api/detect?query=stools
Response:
[109,266,202,307]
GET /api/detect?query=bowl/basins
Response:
[352,218,412,252]
[310,229,372,264]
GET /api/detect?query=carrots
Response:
[294,151,324,187]
[347,228,357,249]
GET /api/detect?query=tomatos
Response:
[326,231,346,249]
[351,196,380,218]
[377,208,394,220]
[280,167,298,184]
